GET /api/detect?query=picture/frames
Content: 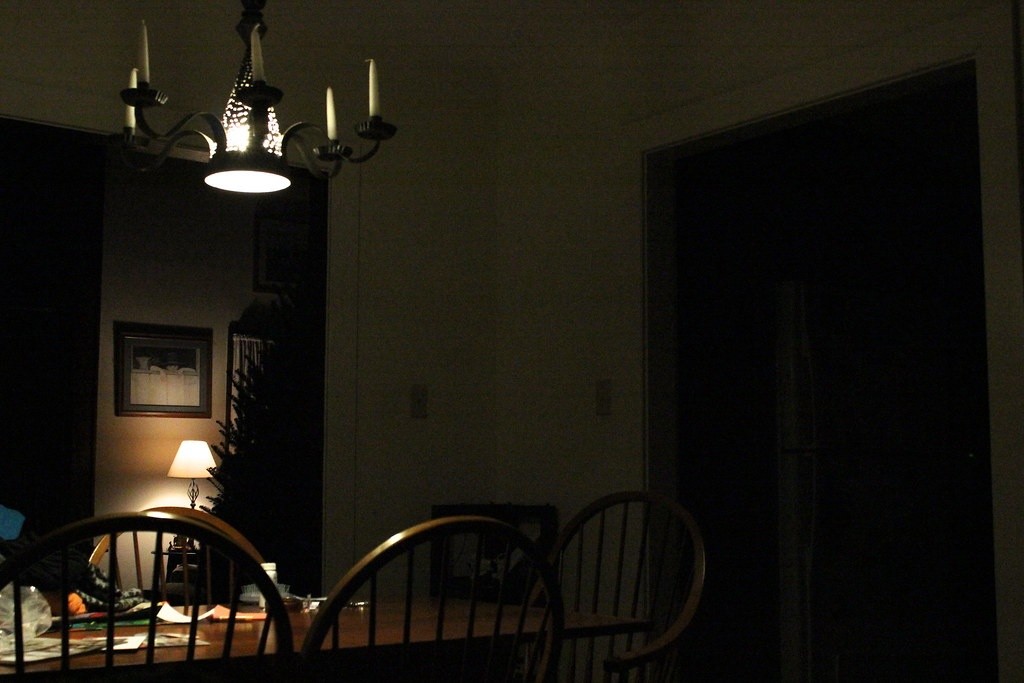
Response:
[253,211,310,294]
[111,319,214,420]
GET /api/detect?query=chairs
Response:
[295,515,565,683]
[0,512,295,683]
[431,504,562,611]
[525,491,708,683]
[87,507,265,606]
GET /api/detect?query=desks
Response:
[0,603,653,683]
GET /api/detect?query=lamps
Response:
[118,0,396,194]
[167,440,217,552]
